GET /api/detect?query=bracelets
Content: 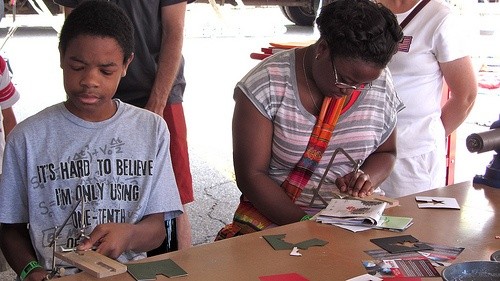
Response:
[302,214,311,223]
[20,259,43,281]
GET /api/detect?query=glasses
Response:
[330,54,372,91]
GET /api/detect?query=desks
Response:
[45,181,500,281]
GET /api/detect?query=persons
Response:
[216,0,404,243]
[363,0,478,203]
[0,0,197,281]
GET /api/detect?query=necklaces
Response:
[303,43,322,112]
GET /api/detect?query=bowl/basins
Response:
[489,250,500,261]
[440,262,500,281]
